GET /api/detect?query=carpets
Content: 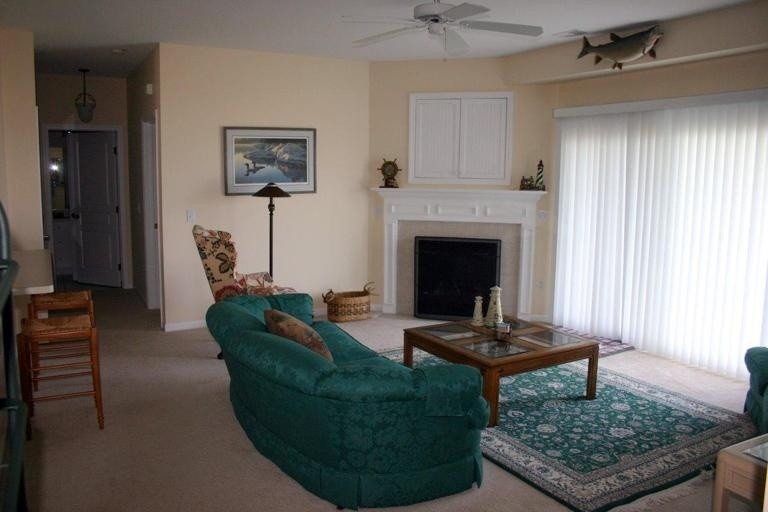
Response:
[529,320,635,358]
[373,346,764,512]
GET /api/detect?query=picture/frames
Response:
[222,121,319,197]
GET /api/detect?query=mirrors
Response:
[49,141,70,218]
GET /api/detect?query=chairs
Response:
[192,222,315,360]
[744,346,768,437]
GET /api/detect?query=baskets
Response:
[322,282,380,323]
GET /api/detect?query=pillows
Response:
[264,308,335,365]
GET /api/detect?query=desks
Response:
[711,433,768,512]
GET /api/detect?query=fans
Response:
[340,0,542,62]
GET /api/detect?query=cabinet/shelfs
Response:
[15,288,106,442]
[7,246,53,346]
[412,97,508,181]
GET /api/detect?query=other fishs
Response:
[576,23,666,73]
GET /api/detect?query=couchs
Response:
[204,291,493,511]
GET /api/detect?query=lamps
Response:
[73,68,97,125]
[251,182,291,279]
